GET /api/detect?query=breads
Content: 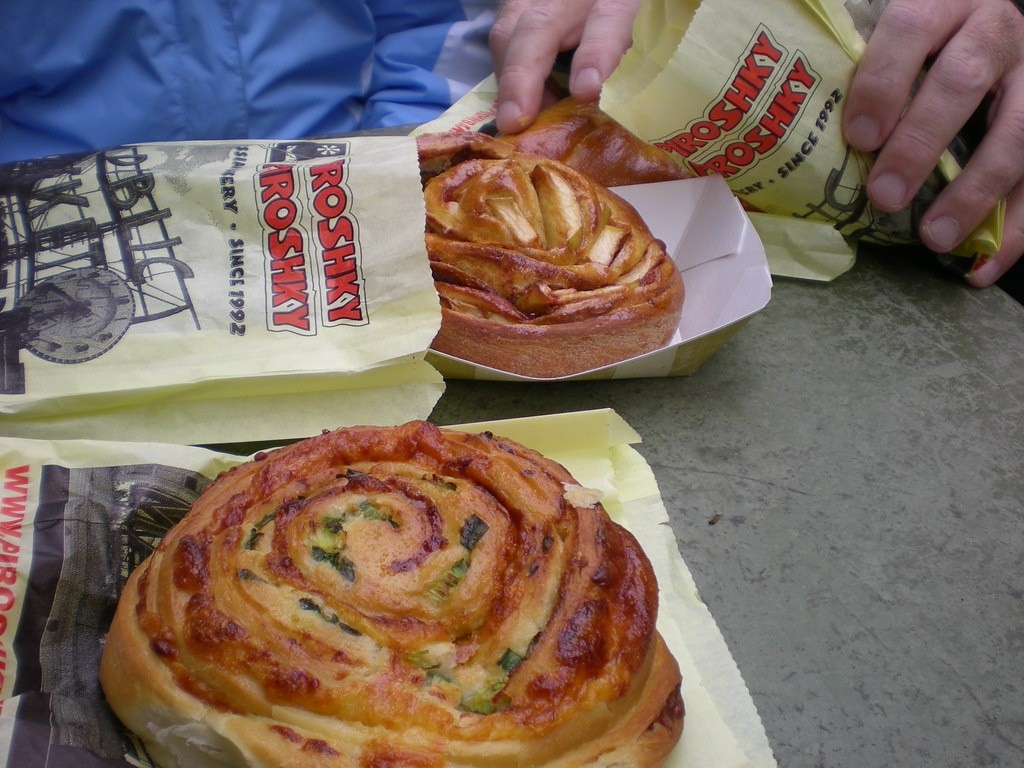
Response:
[103,419,684,767]
[423,160,684,379]
[492,96,690,187]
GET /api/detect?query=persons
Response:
[489,0,1024,291]
